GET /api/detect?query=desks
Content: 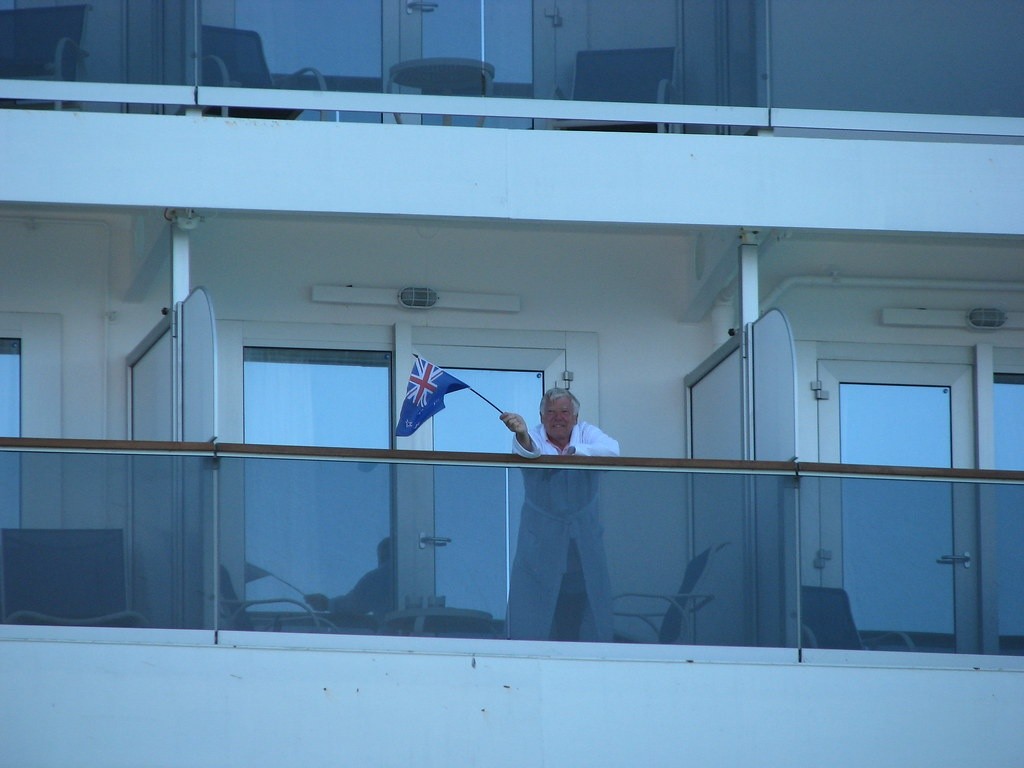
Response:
[388,56,495,128]
[383,607,504,639]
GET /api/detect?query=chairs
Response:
[218,565,343,635]
[0,4,91,111]
[611,540,720,644]
[199,25,329,122]
[0,528,156,628]
[800,585,916,652]
[547,48,680,133]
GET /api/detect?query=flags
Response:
[392,357,473,441]
[246,562,269,584]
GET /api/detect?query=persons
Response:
[502,388,621,643]
[305,535,397,615]
